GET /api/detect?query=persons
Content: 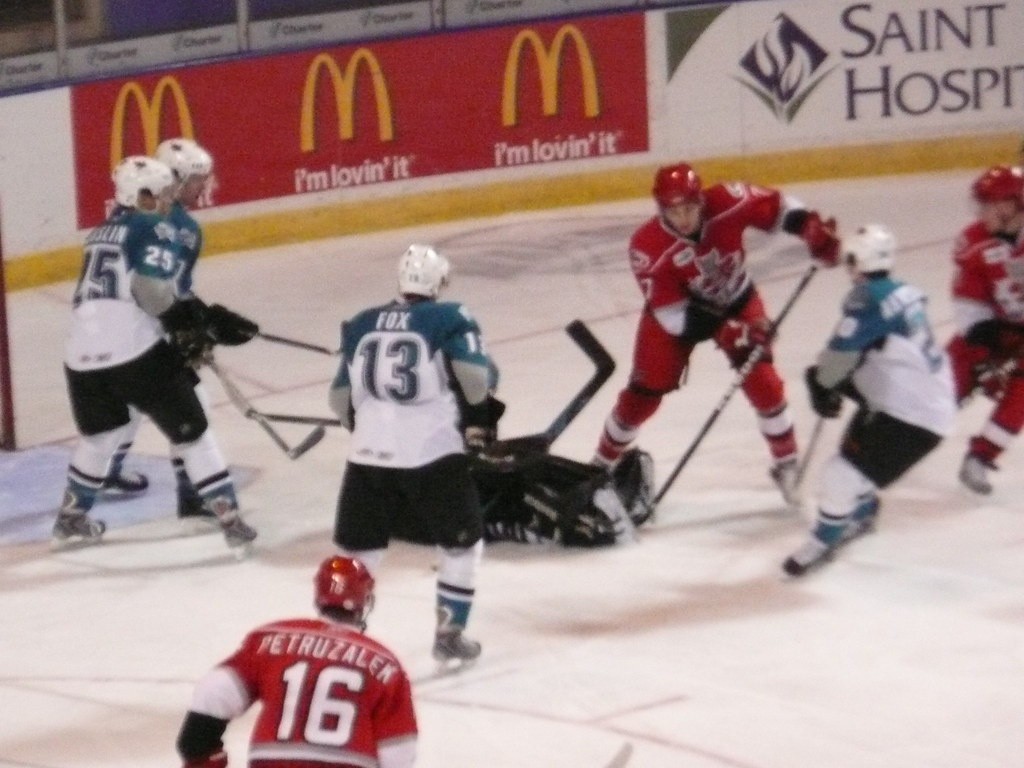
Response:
[470,428,656,547]
[592,159,844,514]
[943,162,1024,495]
[329,243,506,672]
[101,139,258,532]
[50,156,259,559]
[175,551,419,768]
[778,223,953,581]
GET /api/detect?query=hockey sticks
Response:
[606,739,635,768]
[427,320,616,573]
[232,316,350,356]
[771,415,824,503]
[199,356,327,461]
[627,262,820,525]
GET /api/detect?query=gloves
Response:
[800,214,839,263]
[456,392,506,457]
[806,364,843,419]
[976,357,1015,405]
[160,297,259,349]
[717,319,757,364]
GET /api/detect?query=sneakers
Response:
[51,488,105,552]
[210,498,258,558]
[769,461,808,512]
[959,437,996,500]
[176,483,219,536]
[102,462,149,501]
[854,491,879,538]
[784,512,849,579]
[430,607,481,669]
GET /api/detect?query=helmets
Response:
[844,224,896,273]
[314,557,374,614]
[112,157,171,210]
[157,139,214,208]
[654,164,701,208]
[399,244,447,299]
[975,165,1023,205]
